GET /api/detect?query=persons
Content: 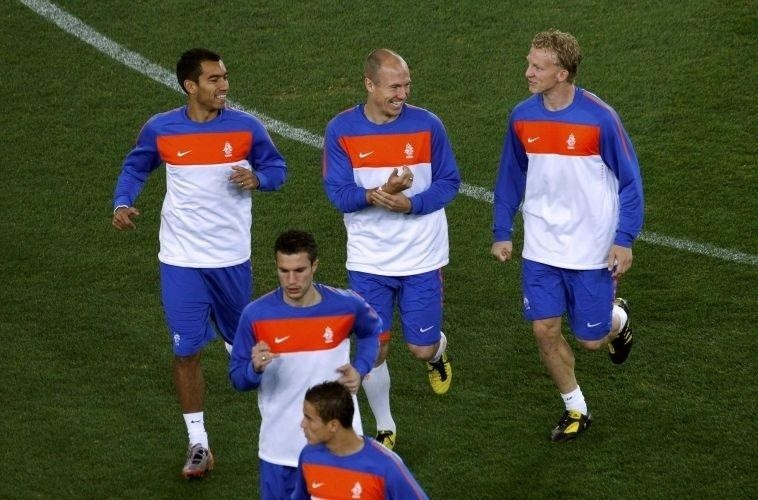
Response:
[320,47,463,456]
[226,225,386,498]
[279,379,431,500]
[488,29,650,442]
[109,48,289,484]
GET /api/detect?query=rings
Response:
[409,177,414,181]
[241,181,244,187]
[260,356,267,361]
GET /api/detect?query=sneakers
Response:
[426,352,453,395]
[607,296,634,365]
[374,428,396,450]
[549,408,594,445]
[180,441,214,479]
